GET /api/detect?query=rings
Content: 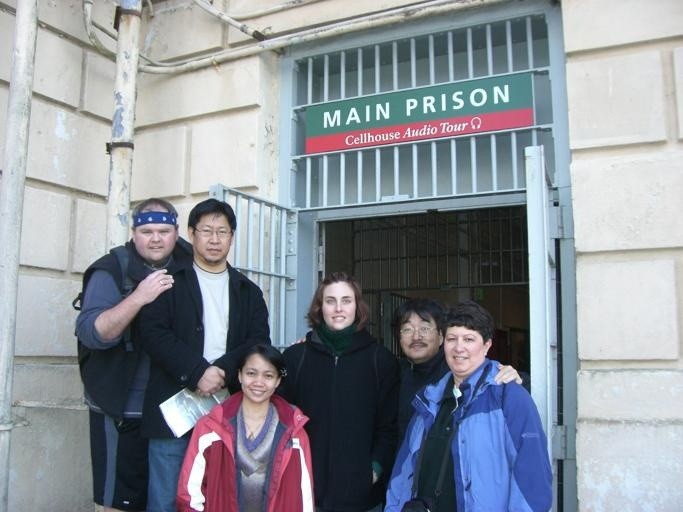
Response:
[159,279,165,285]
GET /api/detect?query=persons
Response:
[279,271,398,512]
[176,344,315,512]
[74,194,189,512]
[388,297,531,445]
[136,199,271,512]
[386,301,555,512]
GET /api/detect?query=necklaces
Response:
[241,417,264,442]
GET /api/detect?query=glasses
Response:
[399,326,439,335]
[194,227,232,237]
